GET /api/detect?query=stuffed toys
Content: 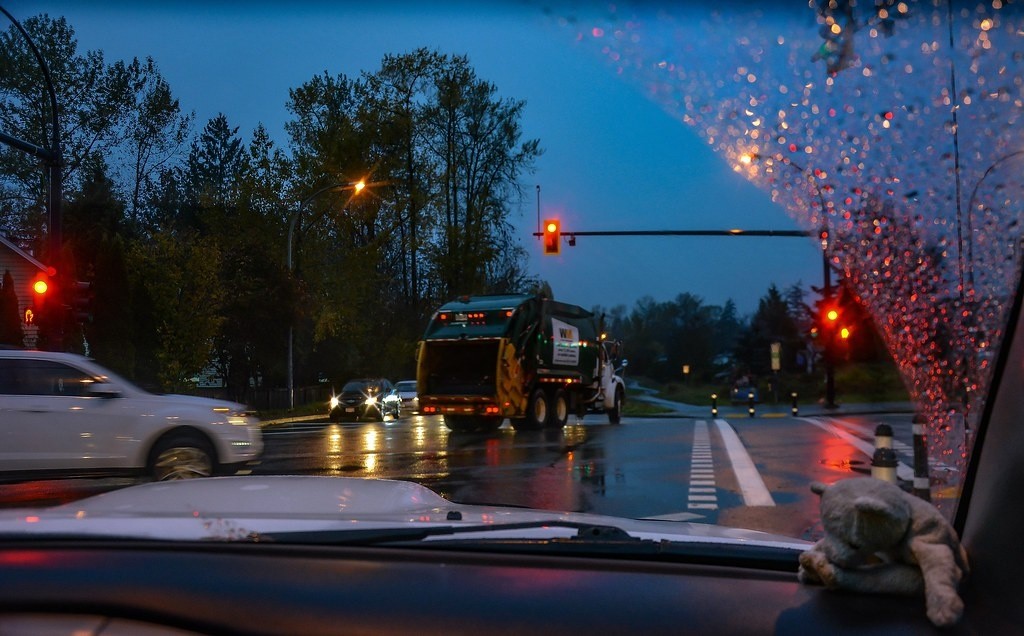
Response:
[797,478,964,627]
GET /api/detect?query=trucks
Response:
[416,294,628,437]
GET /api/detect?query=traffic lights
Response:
[32,279,51,297]
[544,218,561,255]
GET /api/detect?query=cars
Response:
[394,380,420,413]
[329,379,400,422]
[0,348,261,482]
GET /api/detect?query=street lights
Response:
[826,309,840,324]
[285,179,367,413]
